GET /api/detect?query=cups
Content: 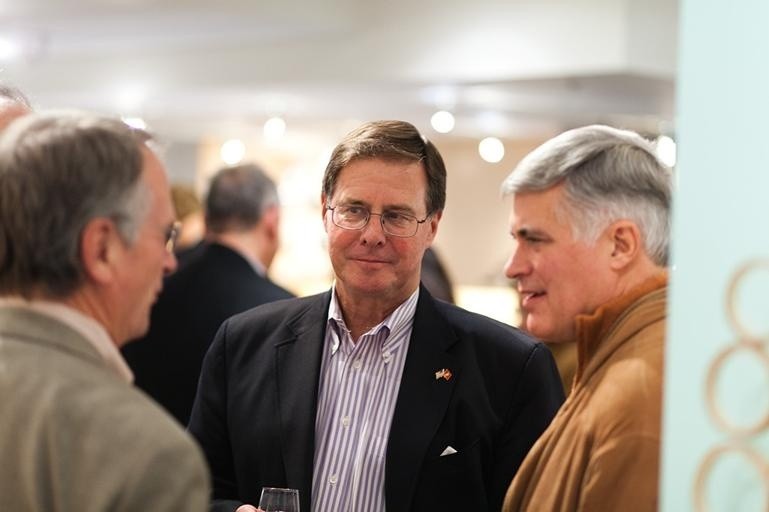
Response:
[257,487,300,512]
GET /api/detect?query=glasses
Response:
[329,202,421,238]
[130,218,182,253]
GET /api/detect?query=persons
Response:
[1,86,35,130]
[0,109,213,512]
[118,162,298,432]
[420,247,455,304]
[186,116,566,512]
[502,121,672,511]
[509,279,579,399]
